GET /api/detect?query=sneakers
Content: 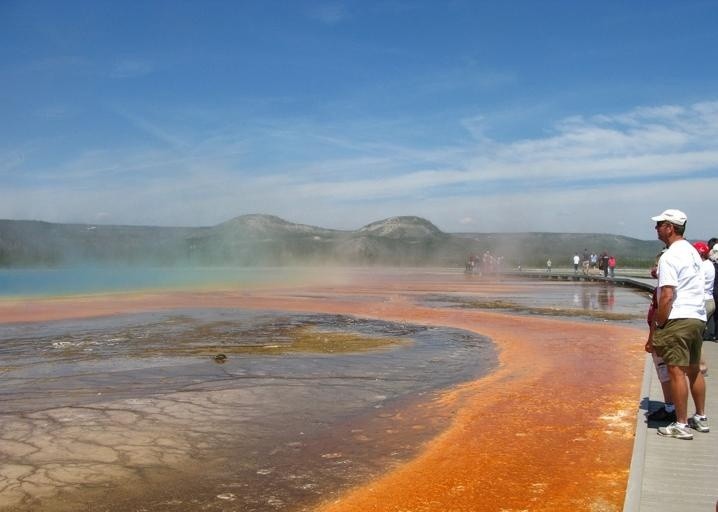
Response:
[648,406,677,422]
[687,414,710,433]
[658,424,695,440]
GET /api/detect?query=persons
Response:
[703,237,718,341]
[546,256,553,271]
[649,209,711,439]
[568,248,616,278]
[646,249,679,423]
[692,240,716,375]
[707,241,718,342]
[461,249,506,274]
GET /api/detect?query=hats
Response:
[693,243,709,253]
[710,244,717,260]
[652,210,688,226]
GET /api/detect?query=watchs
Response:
[652,320,664,329]
